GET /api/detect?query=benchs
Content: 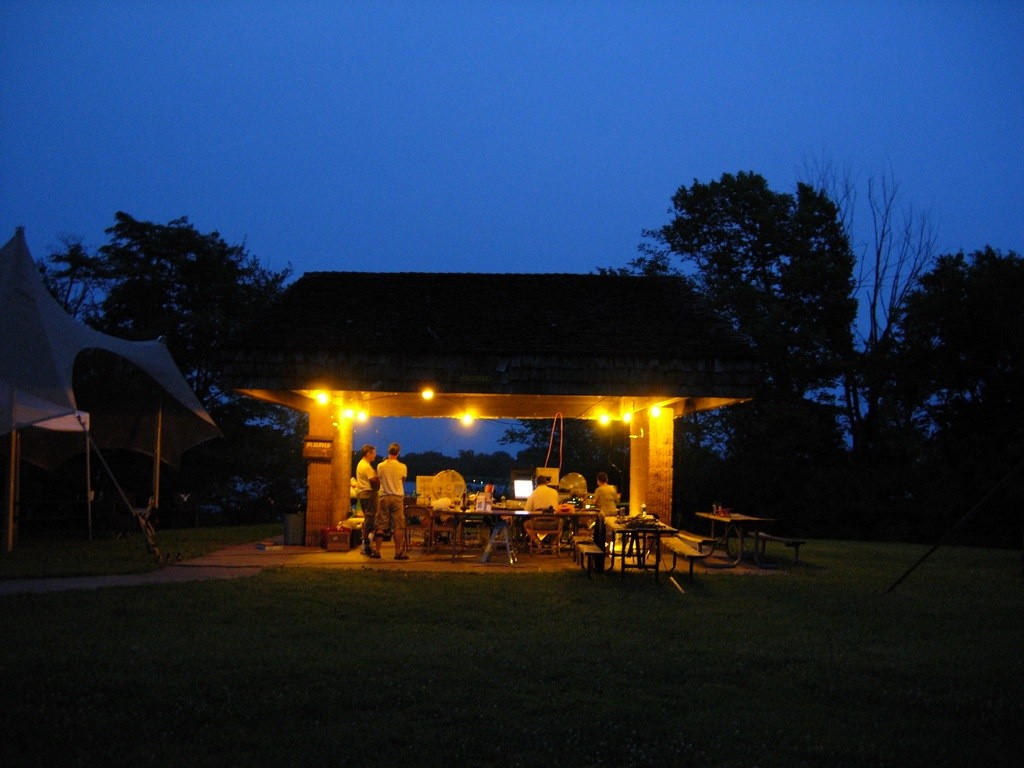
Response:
[661,536,707,585]
[746,531,805,568]
[675,529,718,567]
[571,536,605,582]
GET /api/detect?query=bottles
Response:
[485,497,491,511]
[641,502,647,516]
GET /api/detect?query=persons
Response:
[356,442,408,560]
[467,484,512,526]
[524,476,562,555]
[592,472,617,556]
[350,472,358,509]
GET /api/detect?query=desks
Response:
[434,509,601,552]
[601,516,679,585]
[696,512,777,569]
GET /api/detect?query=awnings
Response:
[0,226,224,566]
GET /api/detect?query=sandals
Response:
[394,550,409,559]
[369,550,381,559]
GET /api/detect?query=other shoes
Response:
[362,539,372,554]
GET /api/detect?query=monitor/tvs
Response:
[513,479,534,499]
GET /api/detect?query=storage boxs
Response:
[322,528,350,551]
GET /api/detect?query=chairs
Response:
[401,505,456,551]
[529,516,562,557]
